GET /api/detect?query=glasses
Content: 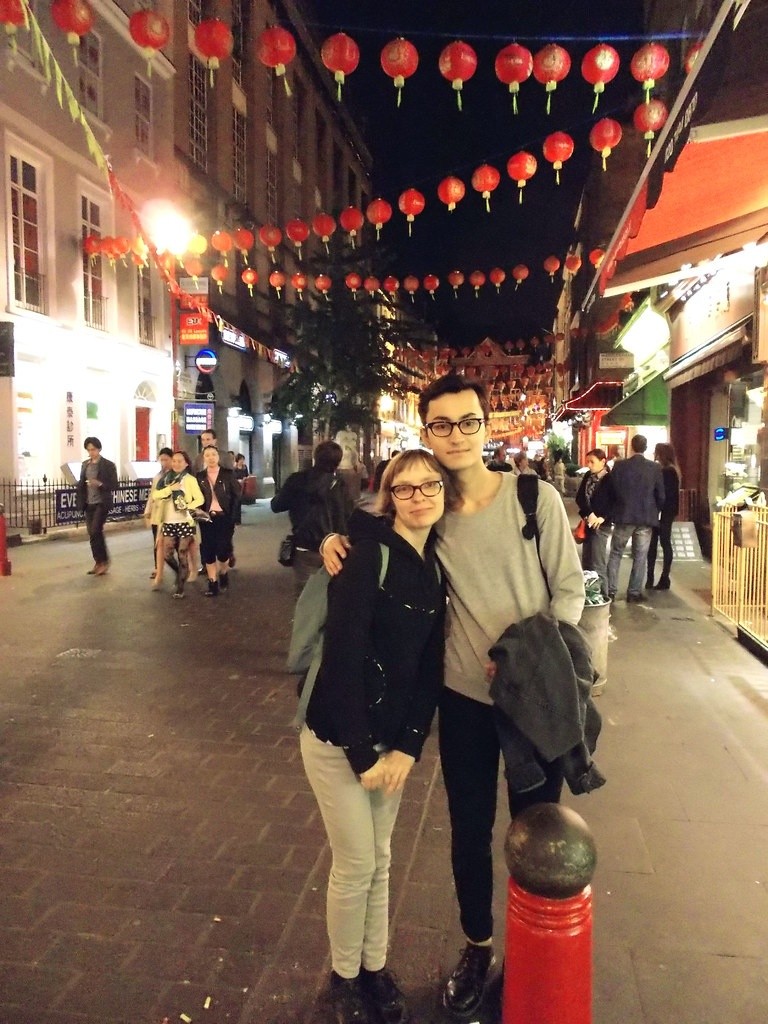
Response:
[388,481,444,501]
[426,418,485,437]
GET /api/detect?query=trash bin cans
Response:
[577,596,612,697]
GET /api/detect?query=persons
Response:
[575,449,612,595]
[229,451,248,480]
[195,446,240,597]
[298,449,456,1024]
[485,447,546,480]
[554,458,565,492]
[270,440,354,598]
[76,437,118,577]
[153,451,205,599]
[144,447,179,578]
[646,443,680,589]
[606,434,665,604]
[191,429,233,476]
[319,375,585,1017]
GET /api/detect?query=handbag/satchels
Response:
[575,519,587,544]
[279,540,295,567]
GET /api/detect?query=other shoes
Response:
[187,571,198,582]
[198,567,206,574]
[173,591,184,599]
[653,583,668,590]
[151,579,163,591]
[87,564,109,575]
[627,594,649,603]
[228,556,236,567]
[149,572,156,578]
[205,579,218,596]
[331,970,366,1024]
[362,968,407,1024]
[218,571,229,592]
[645,581,654,589]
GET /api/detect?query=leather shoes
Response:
[442,941,495,1018]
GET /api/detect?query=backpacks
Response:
[286,543,442,673]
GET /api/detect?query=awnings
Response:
[600,367,669,427]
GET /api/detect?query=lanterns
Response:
[381,327,579,411]
[84,98,668,304]
[0,0,702,106]
